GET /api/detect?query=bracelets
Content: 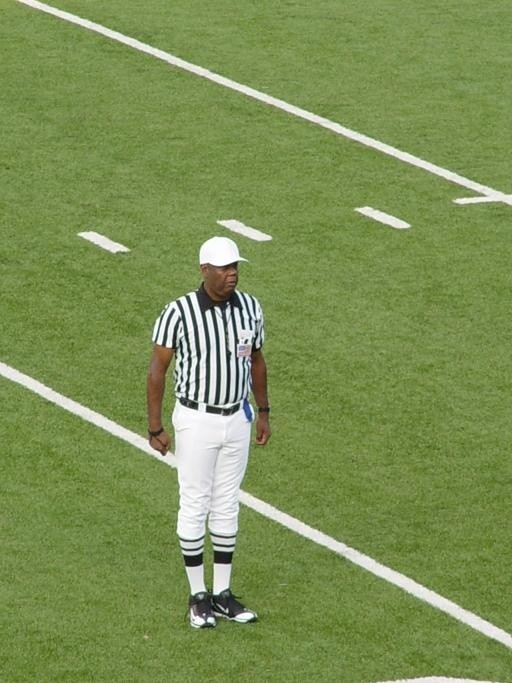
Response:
[145,427,164,436]
[256,405,271,414]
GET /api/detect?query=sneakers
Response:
[209,587,258,624]
[188,590,216,628]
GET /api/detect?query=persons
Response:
[145,234,273,630]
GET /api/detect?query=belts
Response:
[179,396,240,416]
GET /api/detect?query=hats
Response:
[198,235,249,267]
